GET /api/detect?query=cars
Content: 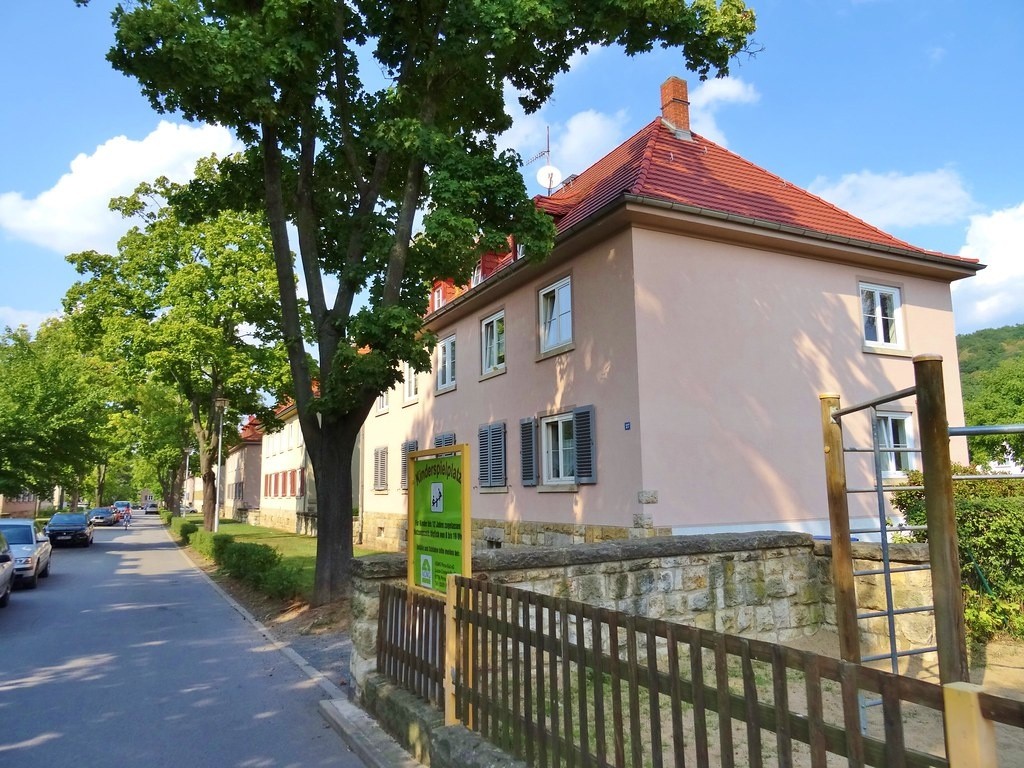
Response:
[0,531,15,608]
[113,501,131,518]
[87,505,121,525]
[145,502,160,514]
[0,517,53,590]
[42,512,95,548]
[131,505,144,510]
[180,505,198,514]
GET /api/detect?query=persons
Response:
[123,506,131,526]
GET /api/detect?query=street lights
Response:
[213,397,231,532]
[183,446,194,516]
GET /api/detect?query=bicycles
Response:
[124,513,132,530]
